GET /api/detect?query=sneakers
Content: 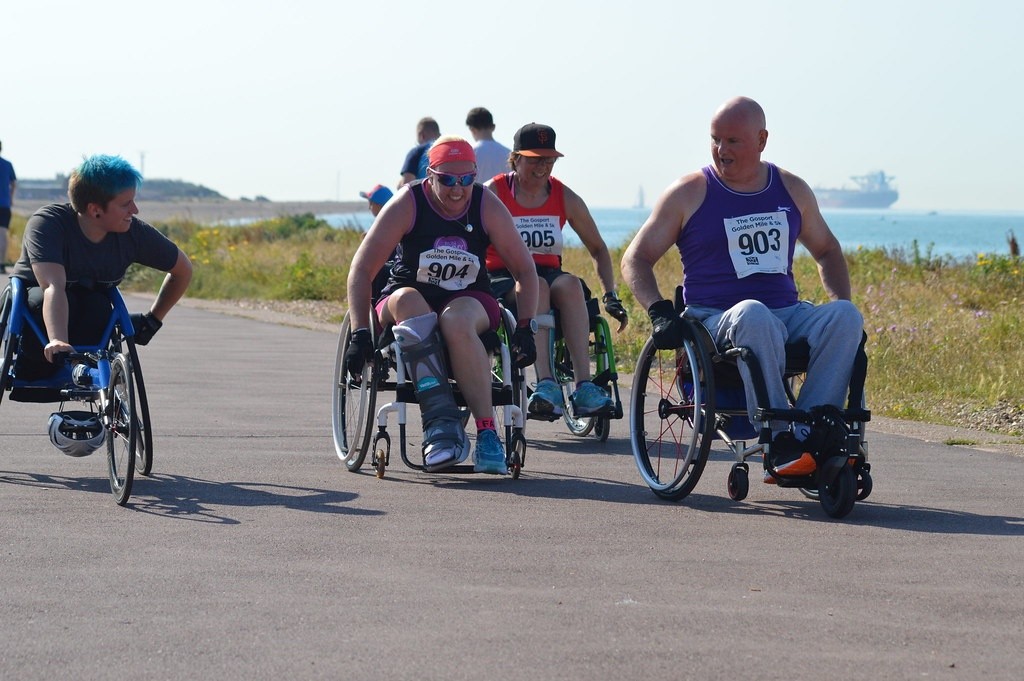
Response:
[763,431,816,484]
[573,382,614,414]
[528,380,565,415]
[472,430,508,475]
[847,447,865,468]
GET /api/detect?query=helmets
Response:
[48,411,105,457]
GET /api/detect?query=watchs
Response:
[603,291,617,298]
[516,318,538,334]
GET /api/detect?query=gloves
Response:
[647,299,692,349]
[130,312,162,345]
[859,330,867,351]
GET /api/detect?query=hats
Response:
[513,123,564,157]
[360,185,393,206]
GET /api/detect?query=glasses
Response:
[516,156,555,164]
[429,165,478,186]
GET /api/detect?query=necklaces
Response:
[428,187,472,232]
[514,174,539,197]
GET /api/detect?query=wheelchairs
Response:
[497,293,623,443]
[629,286,875,517]
[332,262,527,480]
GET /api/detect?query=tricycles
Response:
[0,276,154,506]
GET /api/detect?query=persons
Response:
[359,185,399,261]
[465,108,513,186]
[621,98,862,483]
[0,142,16,273]
[0,154,193,389]
[482,123,629,418]
[345,135,541,475]
[396,118,441,189]
